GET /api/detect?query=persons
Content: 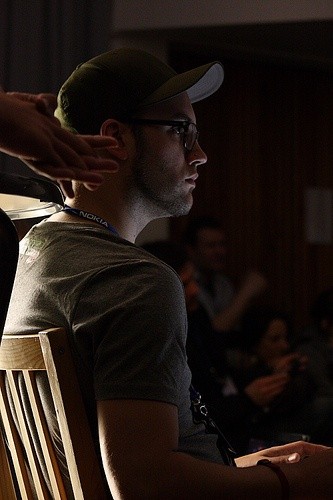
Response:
[0,46,333,500]
[138,218,333,457]
[0,92,121,201]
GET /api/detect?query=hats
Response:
[53,46,224,134]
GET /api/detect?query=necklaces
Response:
[60,205,119,234]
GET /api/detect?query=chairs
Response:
[0,326,107,500]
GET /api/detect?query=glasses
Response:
[127,118,200,152]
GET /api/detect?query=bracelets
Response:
[257,459,291,500]
